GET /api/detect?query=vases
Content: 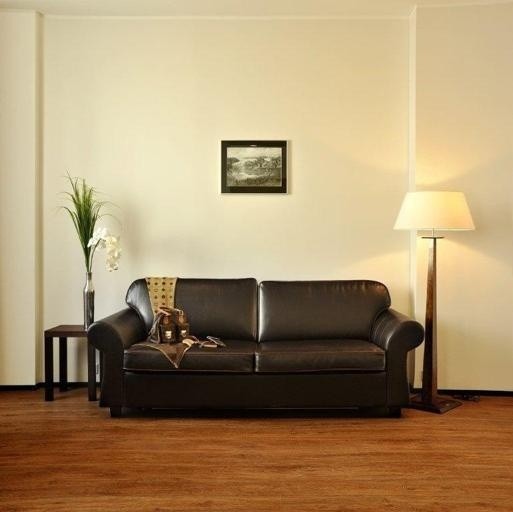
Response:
[83,272,95,331]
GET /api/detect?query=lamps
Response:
[392,191,476,414]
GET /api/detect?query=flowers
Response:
[59,170,122,272]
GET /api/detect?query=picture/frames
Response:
[221,140,287,193]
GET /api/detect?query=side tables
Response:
[45,325,102,400]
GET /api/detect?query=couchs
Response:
[87,279,425,419]
